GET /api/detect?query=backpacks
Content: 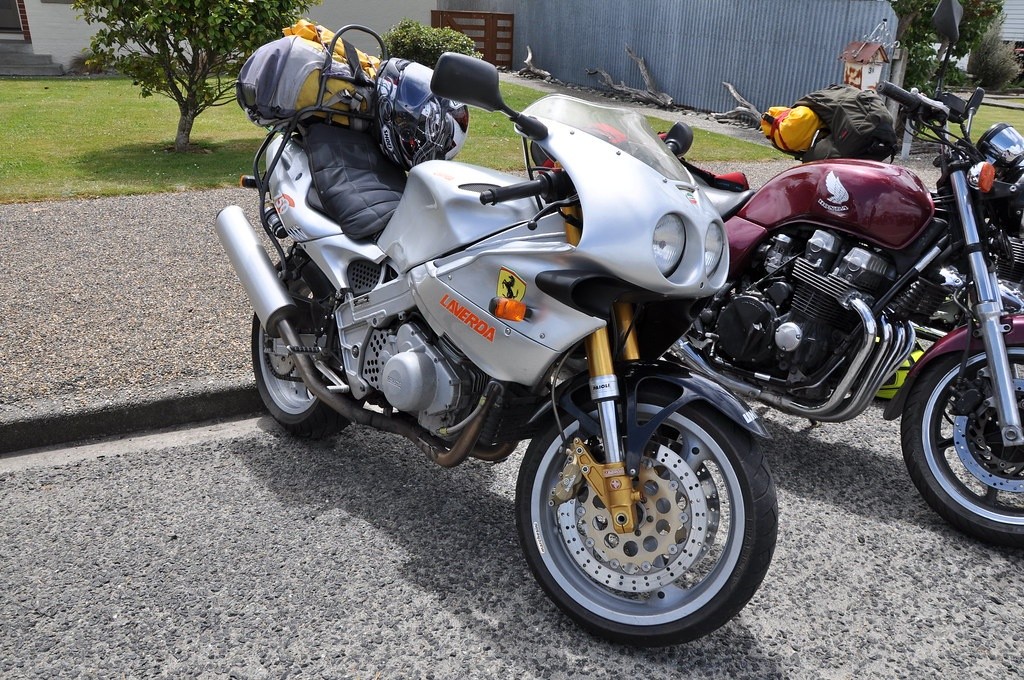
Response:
[796,82,900,165]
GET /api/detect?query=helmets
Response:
[376,57,470,171]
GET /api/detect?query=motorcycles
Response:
[215,25,779,645]
[658,1,1024,547]
